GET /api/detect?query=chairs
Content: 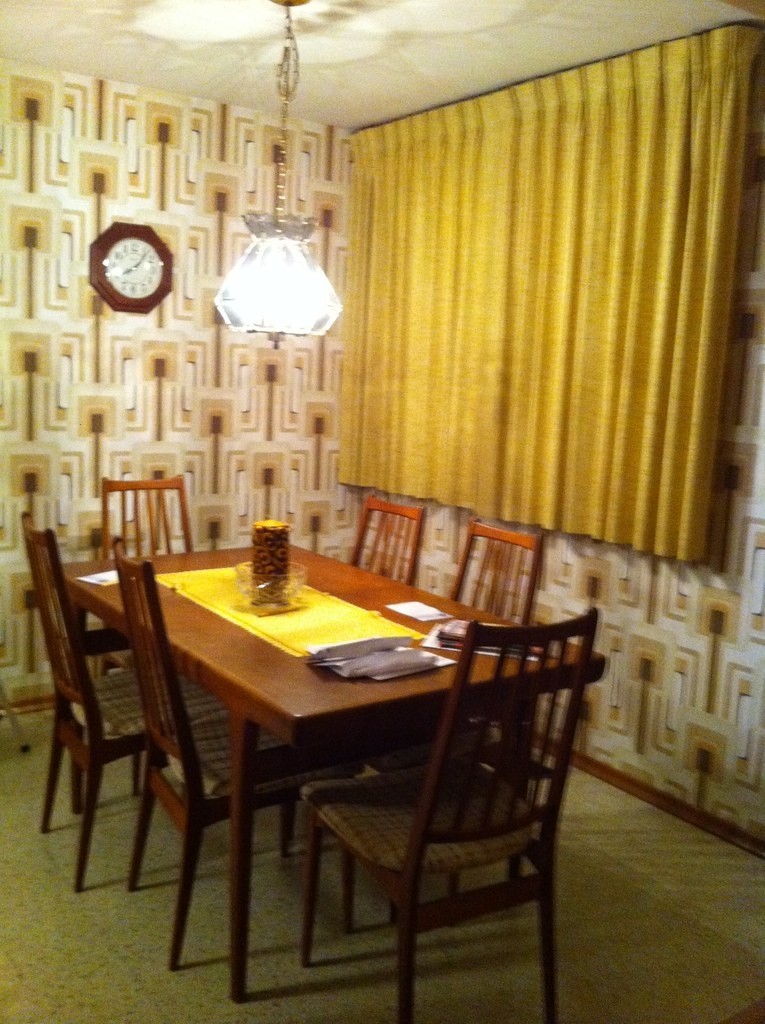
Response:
[447,517,546,622]
[303,603,601,1024]
[348,496,427,586]
[111,535,357,973]
[20,514,228,894]
[94,474,195,674]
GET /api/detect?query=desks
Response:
[68,542,610,1004]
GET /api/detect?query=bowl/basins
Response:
[236,562,309,606]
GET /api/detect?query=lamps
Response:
[212,0,346,352]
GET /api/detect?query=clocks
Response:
[88,221,174,315]
